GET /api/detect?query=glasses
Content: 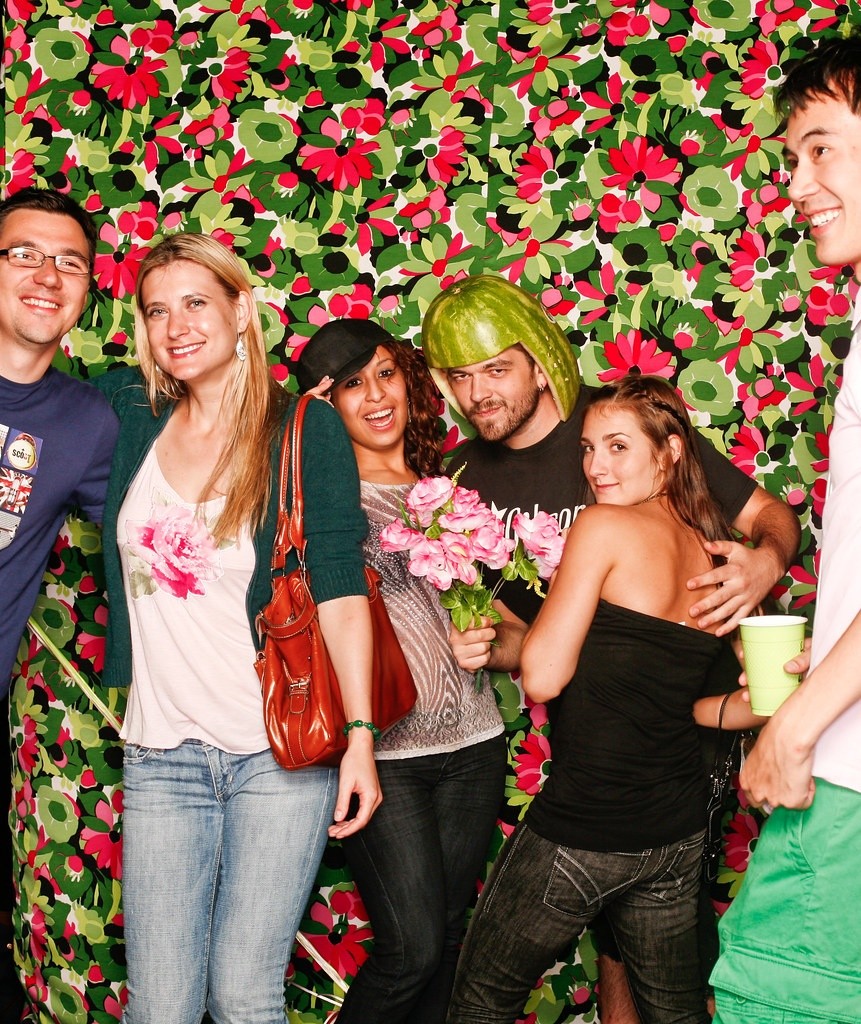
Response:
[0,246,93,277]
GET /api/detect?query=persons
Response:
[708,35,859,1024]
[101,232,382,1024]
[1,185,122,1024]
[423,273,800,1024]
[296,316,507,1024]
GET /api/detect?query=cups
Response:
[737,615,808,717]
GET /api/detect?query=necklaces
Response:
[640,493,666,503]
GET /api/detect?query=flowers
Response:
[378,461,564,697]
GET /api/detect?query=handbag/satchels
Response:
[252,394,418,773]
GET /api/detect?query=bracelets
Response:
[343,720,380,741]
